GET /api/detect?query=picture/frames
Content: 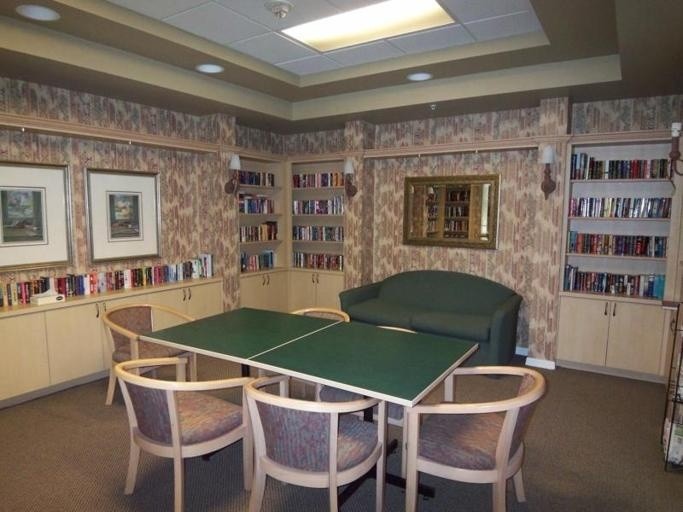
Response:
[82,166,161,265]
[0,159,72,274]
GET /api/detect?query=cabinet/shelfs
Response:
[45,296,152,387]
[425,183,481,239]
[239,157,290,313]
[0,308,48,411]
[290,160,344,309]
[559,136,682,377]
[150,284,226,335]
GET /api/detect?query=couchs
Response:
[339,270,523,379]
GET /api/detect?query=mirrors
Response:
[405,176,498,249]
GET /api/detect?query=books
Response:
[239,170,277,271]
[293,172,344,271]
[0,253,214,306]
[425,186,469,239]
[563,152,672,300]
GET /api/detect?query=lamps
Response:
[540,146,557,200]
[345,160,357,196]
[667,123,683,186]
[224,153,241,194]
